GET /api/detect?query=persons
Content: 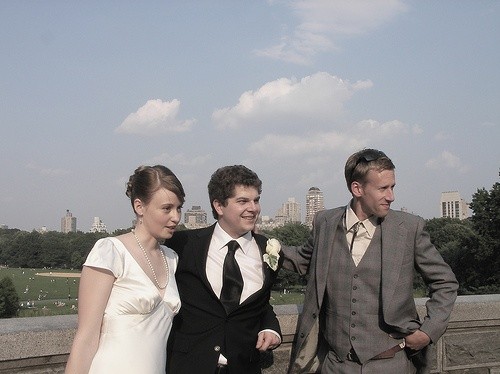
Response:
[64,164,186,374]
[254,148,459,374]
[164,164,285,374]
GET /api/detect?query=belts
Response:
[347,342,406,362]
[217,363,228,373]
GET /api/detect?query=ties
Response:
[219,240,244,359]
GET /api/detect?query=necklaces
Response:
[131,228,169,290]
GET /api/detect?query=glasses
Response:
[350,151,386,181]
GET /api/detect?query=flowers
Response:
[263,239,281,271]
